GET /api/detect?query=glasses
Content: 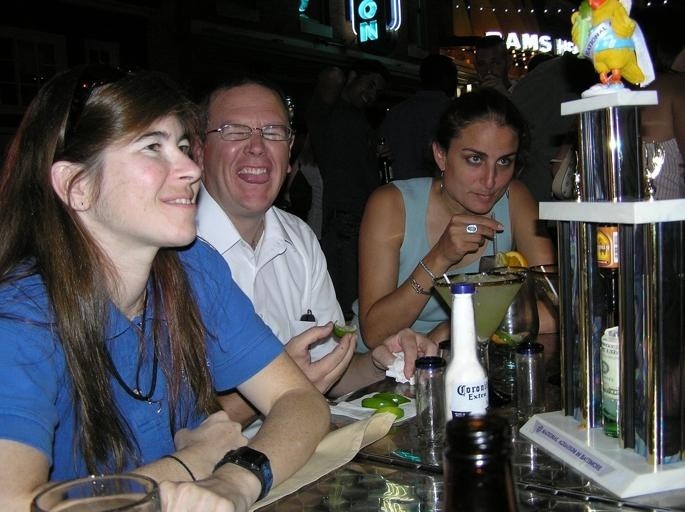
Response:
[203,124,293,142]
[64,65,134,160]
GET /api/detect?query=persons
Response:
[342,86,561,354]
[271,0,685,324]
[179,60,442,430]
[0,64,336,512]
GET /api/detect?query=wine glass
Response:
[532,262,560,310]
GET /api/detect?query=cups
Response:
[432,264,548,422]
[27,472,163,512]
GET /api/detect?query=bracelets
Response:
[162,452,197,484]
[212,446,273,503]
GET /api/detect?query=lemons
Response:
[333,320,357,338]
[495,253,508,266]
[505,251,528,267]
[361,398,398,408]
[372,406,404,418]
[373,393,411,404]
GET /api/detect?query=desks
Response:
[242,333,685,512]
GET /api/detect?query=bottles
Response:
[375,134,396,184]
[413,355,447,467]
[429,283,491,420]
[446,414,511,511]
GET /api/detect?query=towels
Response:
[243,408,397,512]
